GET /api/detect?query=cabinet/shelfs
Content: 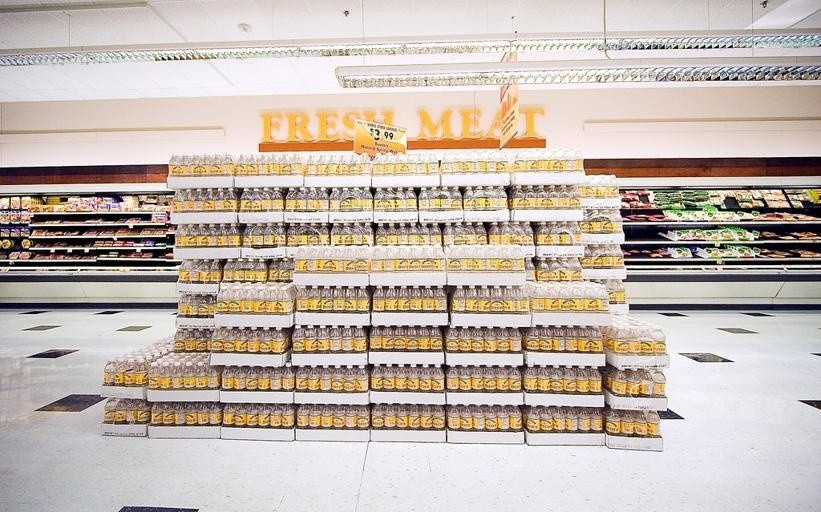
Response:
[0,183,182,303]
[618,175,821,306]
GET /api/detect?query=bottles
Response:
[523,256,585,284]
[164,147,582,175]
[170,183,583,214]
[577,173,627,304]
[599,314,667,439]
[102,336,295,430]
[294,244,525,274]
[174,221,583,248]
[173,259,292,356]
[292,281,610,434]
